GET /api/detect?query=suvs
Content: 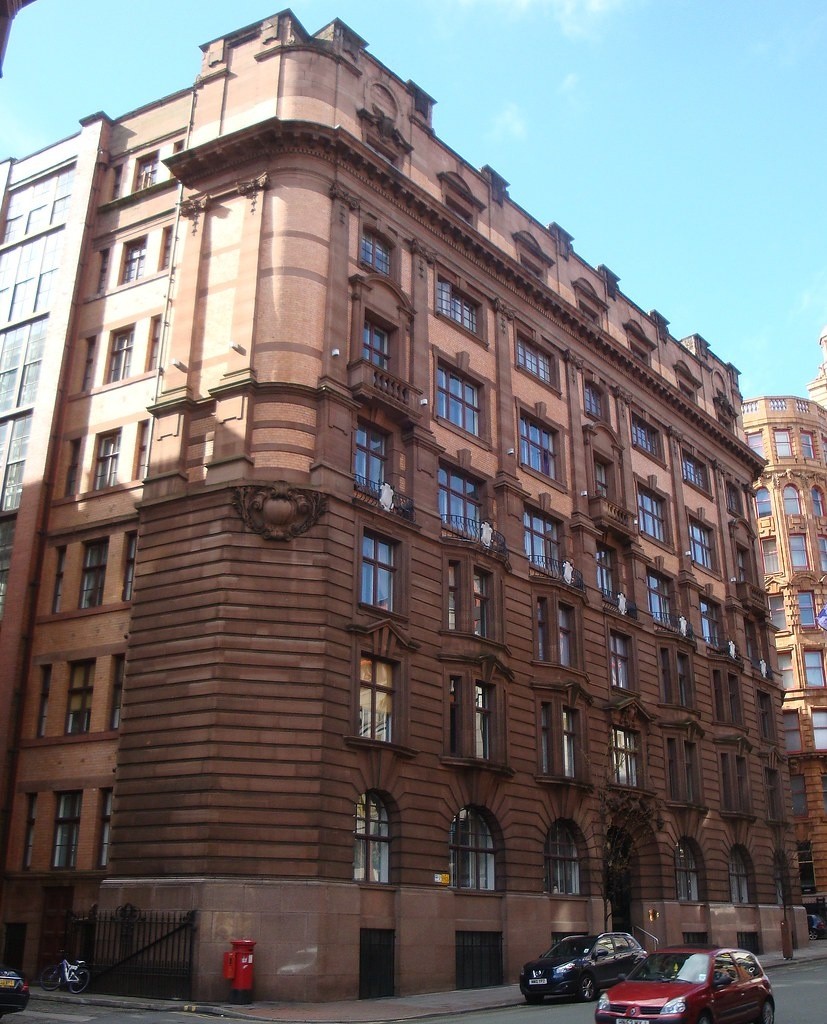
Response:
[519,930,649,1003]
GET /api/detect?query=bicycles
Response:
[39,949,91,995]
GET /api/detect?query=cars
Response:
[807,912,827,941]
[0,967,30,1019]
[593,944,778,1023]
